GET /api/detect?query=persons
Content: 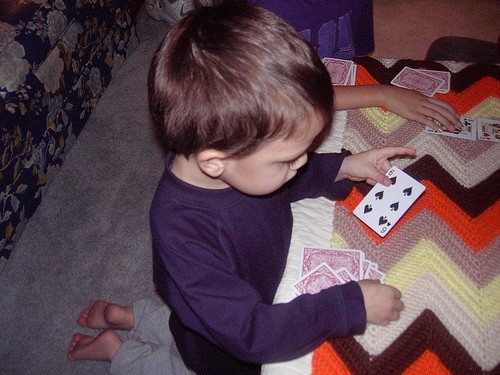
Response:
[327,85,466,134]
[67,1,417,373]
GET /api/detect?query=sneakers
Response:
[144,0,196,28]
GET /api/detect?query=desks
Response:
[257,52,500,375]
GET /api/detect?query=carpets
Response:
[4,16,168,375]
